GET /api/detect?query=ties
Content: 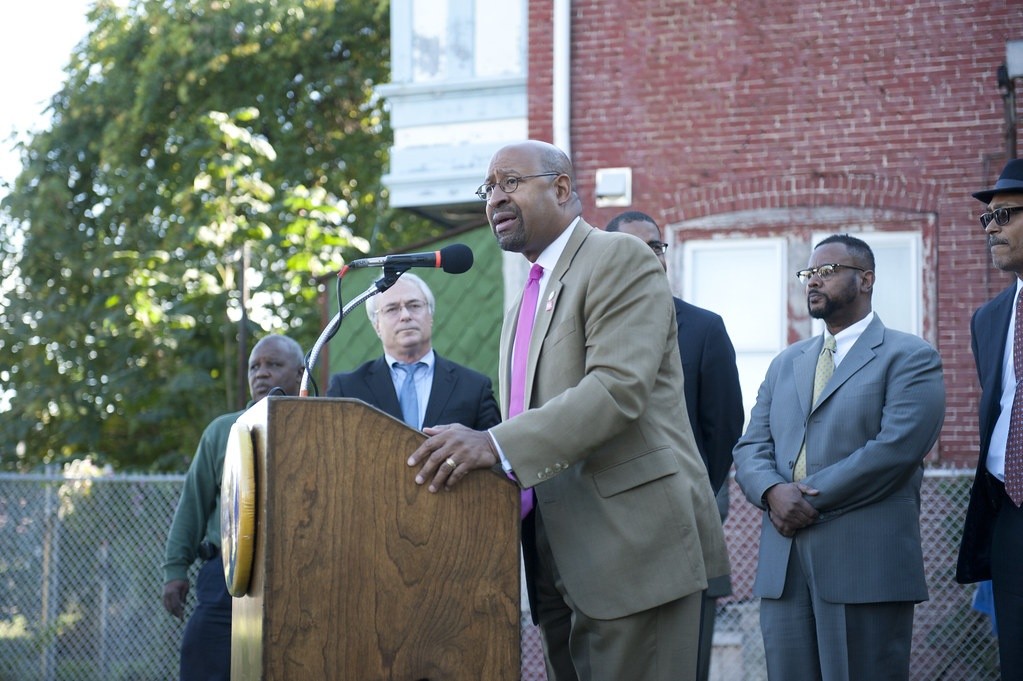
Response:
[793,336,837,482]
[505,264,544,521]
[392,362,424,431]
[1004,287,1022,507]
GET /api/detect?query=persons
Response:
[732,235,946,681]
[956,159,1023,681]
[604,211,746,494]
[325,272,502,438]
[162,334,304,681]
[408,141,731,681]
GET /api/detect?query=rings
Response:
[446,458,457,469]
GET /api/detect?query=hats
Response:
[972,159,1023,204]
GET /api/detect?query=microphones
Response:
[351,243,473,274]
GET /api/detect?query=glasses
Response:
[980,206,1023,229]
[796,264,864,284]
[475,174,559,201]
[650,243,667,255]
[374,300,427,318]
